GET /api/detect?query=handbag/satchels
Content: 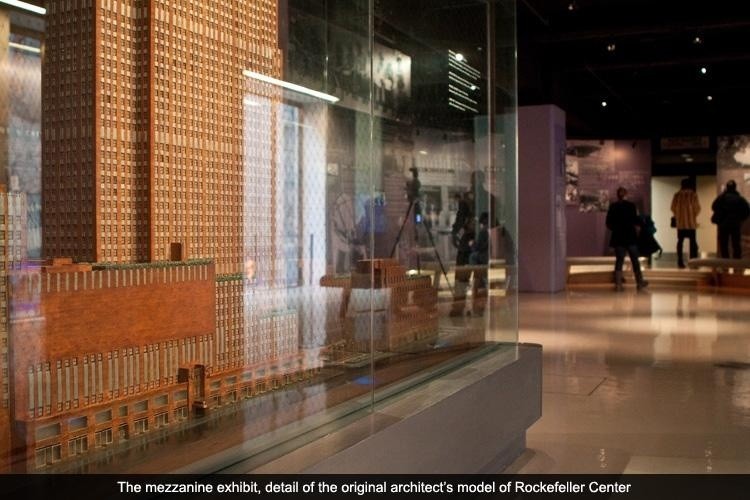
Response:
[671,216,678,226]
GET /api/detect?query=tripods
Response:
[388,190,455,301]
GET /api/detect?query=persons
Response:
[672,178,702,268]
[606,187,662,292]
[711,180,749,259]
[422,169,515,319]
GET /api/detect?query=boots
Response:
[615,271,625,292]
[634,271,648,289]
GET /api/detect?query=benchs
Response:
[687,258,750,286]
[320,273,431,320]
